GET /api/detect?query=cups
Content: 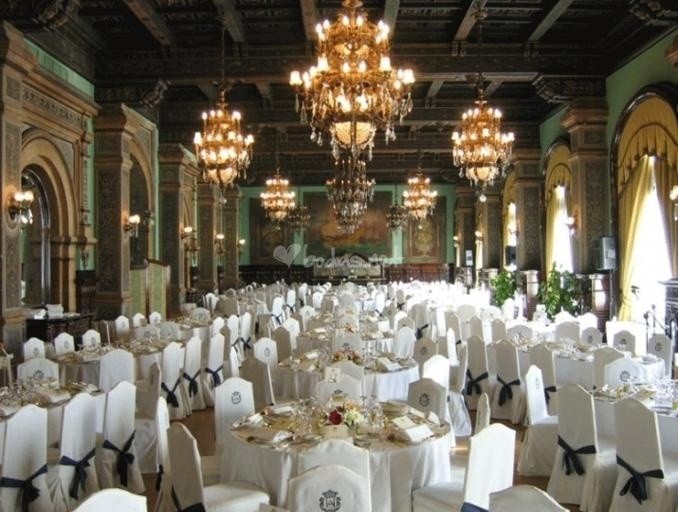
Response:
[559,336,627,360]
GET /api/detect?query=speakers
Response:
[594,237,616,271]
[505,246,516,265]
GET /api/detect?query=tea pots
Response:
[654,374,676,413]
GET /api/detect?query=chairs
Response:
[1,281,678,511]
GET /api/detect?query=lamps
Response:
[191,16,254,191]
[289,0,415,162]
[385,174,406,233]
[261,130,296,223]
[123,214,140,238]
[215,234,225,243]
[450,3,516,203]
[324,161,376,233]
[8,189,35,227]
[403,133,438,223]
[181,226,192,240]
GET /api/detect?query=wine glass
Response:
[291,393,385,441]
[116,333,177,349]
[4,343,114,406]
[313,323,376,373]
